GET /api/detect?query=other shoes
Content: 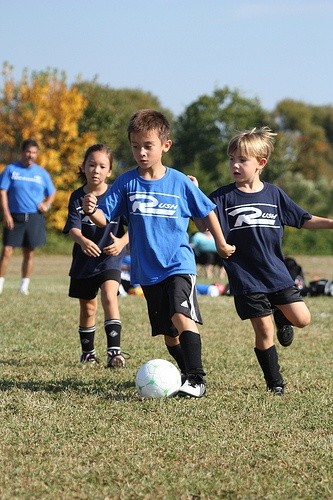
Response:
[19,288,28,296]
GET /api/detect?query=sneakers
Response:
[107,350,131,367]
[80,353,99,364]
[178,375,206,398]
[266,383,287,396]
[274,308,294,346]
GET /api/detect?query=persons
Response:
[82,113,333,398]
[62,145,130,368]
[0,140,57,295]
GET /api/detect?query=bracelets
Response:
[45,201,51,209]
[83,207,96,216]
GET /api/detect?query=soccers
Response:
[134,359,183,400]
